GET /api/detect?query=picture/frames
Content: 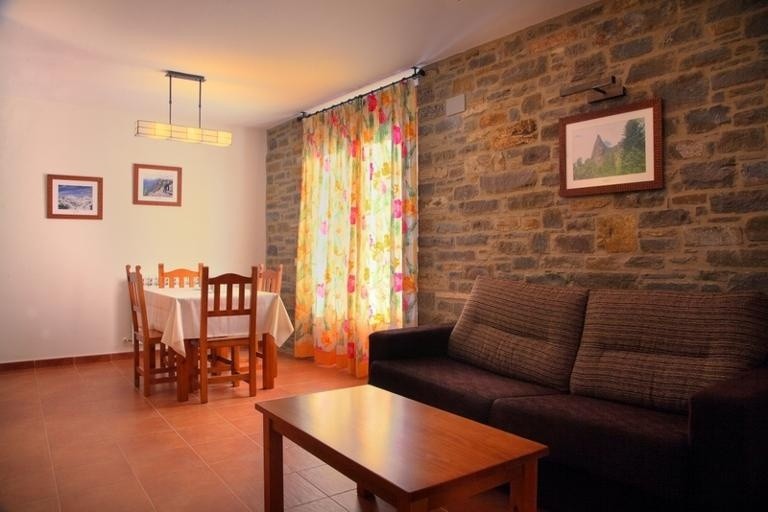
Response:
[133,163,182,207]
[47,173,103,219]
[558,96,663,196]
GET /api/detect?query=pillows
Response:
[567,288,768,412]
[444,276,590,394]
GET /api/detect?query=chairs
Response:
[126,262,284,404]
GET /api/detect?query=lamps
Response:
[133,70,232,148]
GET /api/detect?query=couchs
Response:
[367,321,768,512]
